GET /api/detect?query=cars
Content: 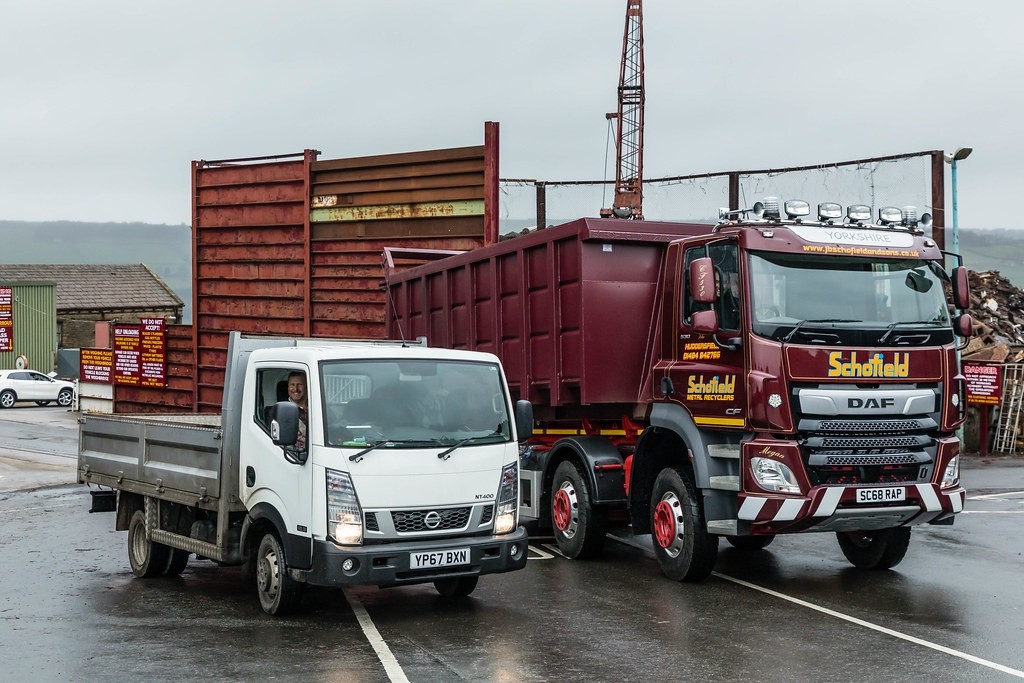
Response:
[0,369,75,408]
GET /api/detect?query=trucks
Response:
[75,329,533,616]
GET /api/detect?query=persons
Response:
[282,371,337,449]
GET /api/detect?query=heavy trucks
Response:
[378,198,974,587]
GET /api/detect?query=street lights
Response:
[942,145,974,377]
